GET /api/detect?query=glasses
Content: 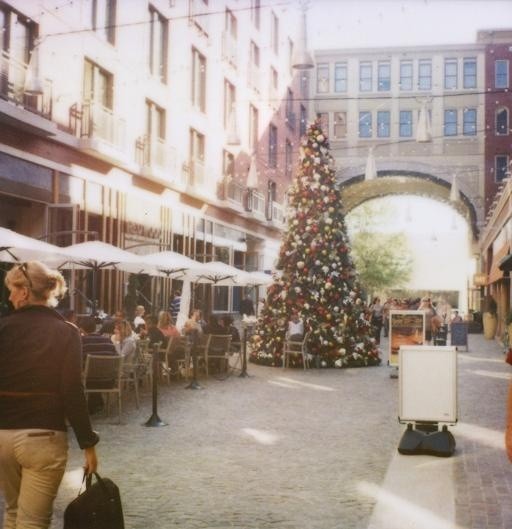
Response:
[19,262,30,285]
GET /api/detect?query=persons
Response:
[452,311,461,321]
[77,304,242,416]
[286,310,304,341]
[367,293,449,343]
[1,261,98,528]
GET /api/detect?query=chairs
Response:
[75,329,247,427]
[280,329,311,371]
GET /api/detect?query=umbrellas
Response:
[0,223,276,321]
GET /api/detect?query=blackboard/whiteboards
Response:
[397,346,458,425]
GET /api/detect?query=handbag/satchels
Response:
[65,470,125,529]
